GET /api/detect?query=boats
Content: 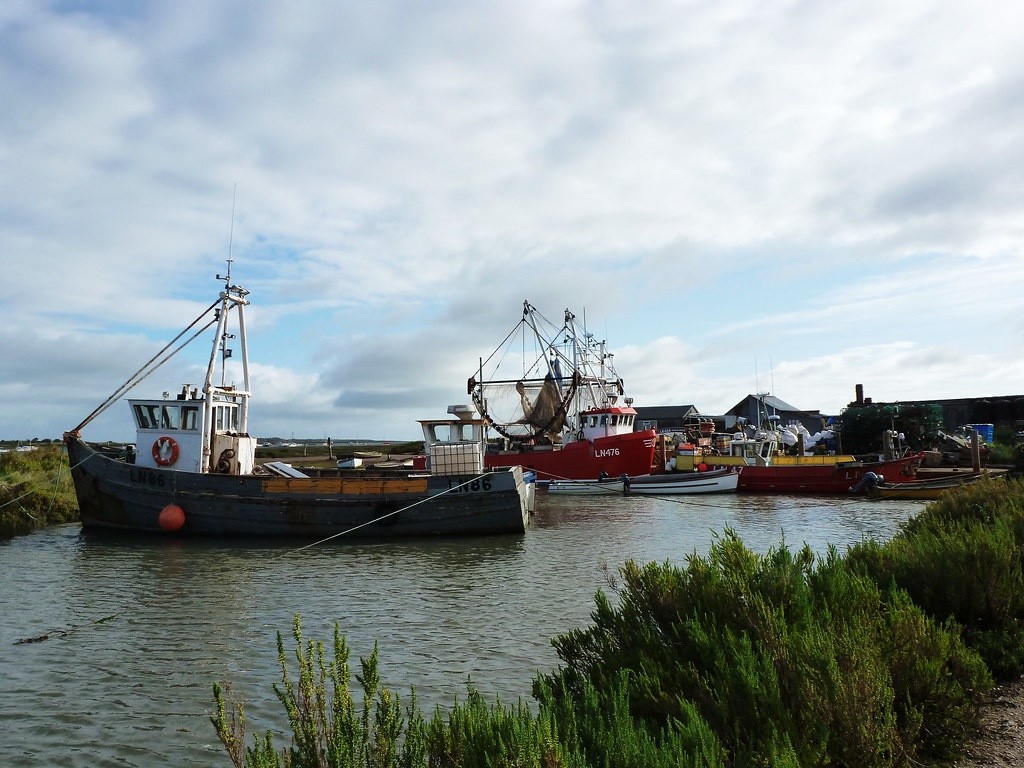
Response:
[62,184,537,545]
[336,457,362,469]
[411,298,1008,501]
[336,454,355,461]
[354,452,382,458]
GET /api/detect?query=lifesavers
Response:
[151,436,180,466]
[252,465,263,475]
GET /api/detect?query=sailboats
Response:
[15,438,38,453]
